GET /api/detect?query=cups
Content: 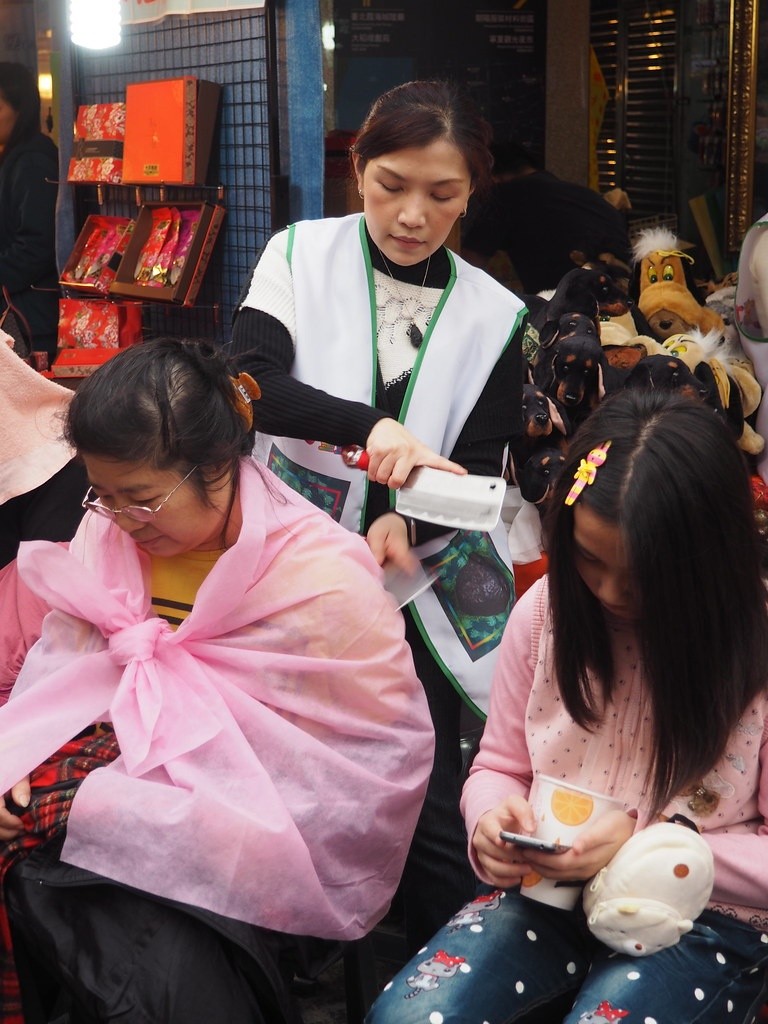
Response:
[520,773,626,911]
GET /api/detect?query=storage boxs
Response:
[122,77,221,187]
[72,100,123,142]
[111,200,223,304]
[53,301,140,378]
[60,215,134,294]
[66,154,122,182]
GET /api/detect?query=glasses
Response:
[82,465,198,522]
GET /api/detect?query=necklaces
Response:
[379,249,430,347]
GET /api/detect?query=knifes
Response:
[341,444,507,532]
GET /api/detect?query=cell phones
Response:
[499,831,570,855]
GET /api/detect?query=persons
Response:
[0,61,59,351]
[0,337,435,1024]
[220,79,631,1024]
[361,383,768,1024]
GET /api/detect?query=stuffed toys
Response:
[600,227,765,456]
